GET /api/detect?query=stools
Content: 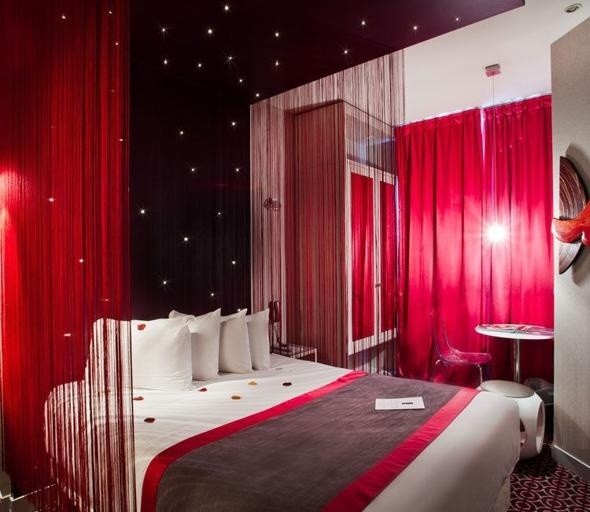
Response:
[476,379,546,460]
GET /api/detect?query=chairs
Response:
[428,315,492,385]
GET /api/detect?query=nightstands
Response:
[270,343,317,362]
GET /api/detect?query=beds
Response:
[43,353,520,511]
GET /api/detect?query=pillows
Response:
[168,307,271,369]
[218,307,255,373]
[84,316,196,390]
[166,307,223,382]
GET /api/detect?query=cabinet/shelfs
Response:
[284,99,400,375]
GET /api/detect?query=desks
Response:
[474,323,554,382]
